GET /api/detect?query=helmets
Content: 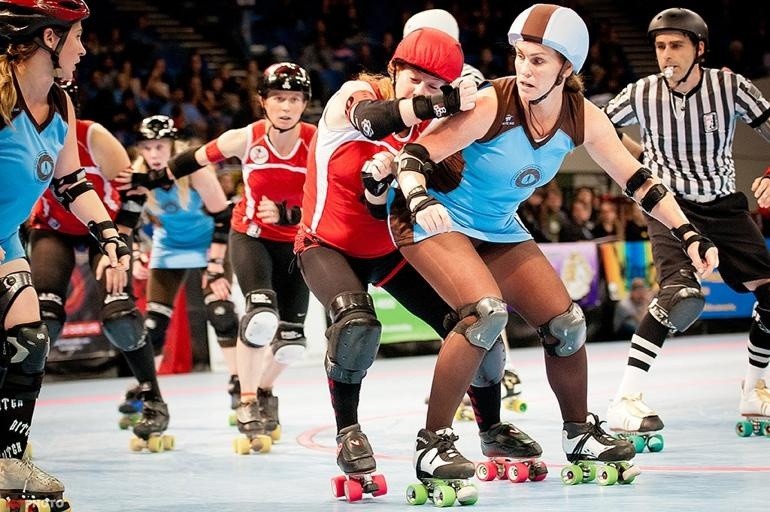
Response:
[138,115,176,139]
[648,8,709,47]
[0,1,89,22]
[391,28,463,83]
[403,8,459,41]
[257,63,312,100]
[508,4,589,74]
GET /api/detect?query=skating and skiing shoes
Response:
[736,378,769,438]
[119,373,282,455]
[1,459,71,512]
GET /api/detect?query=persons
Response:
[19,72,171,436]
[402,9,525,398]
[291,25,544,475]
[522,180,655,245]
[385,2,722,478]
[612,276,654,342]
[579,1,634,98]
[595,7,770,433]
[118,115,242,415]
[1,0,134,492]
[114,62,319,434]
[450,1,518,81]
[69,0,401,138]
